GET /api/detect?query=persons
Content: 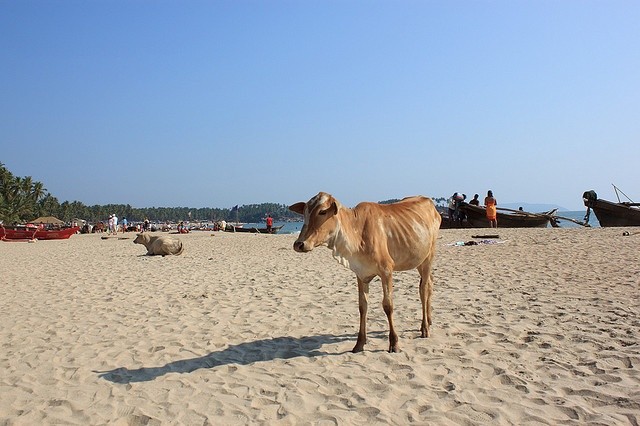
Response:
[484,190,498,229]
[449,192,458,223]
[266,213,274,231]
[106,214,129,235]
[470,194,480,208]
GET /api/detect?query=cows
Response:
[289,192,442,354]
[134,230,185,256]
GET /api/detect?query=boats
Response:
[0,225,80,240]
[456,201,557,228]
[585,184,640,227]
[225,224,285,233]
